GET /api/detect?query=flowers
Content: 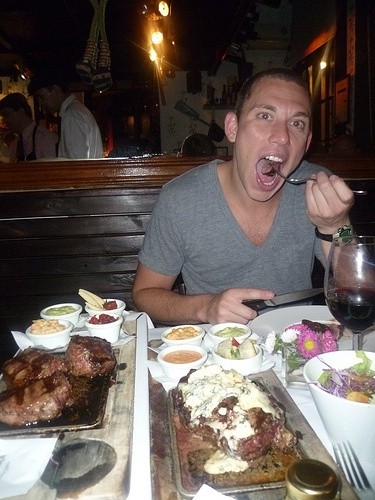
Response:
[266,321,334,362]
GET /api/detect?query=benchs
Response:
[0,155,375,372]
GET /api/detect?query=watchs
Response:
[315,223,353,248]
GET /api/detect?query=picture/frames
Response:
[334,73,351,128]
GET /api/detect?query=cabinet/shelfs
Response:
[88,90,160,157]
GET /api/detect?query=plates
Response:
[249,305,375,358]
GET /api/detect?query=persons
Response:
[0,69,104,164]
[134,67,375,326]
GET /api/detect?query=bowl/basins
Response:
[25,319,74,349]
[161,325,205,346]
[211,340,262,375]
[207,322,251,342]
[84,299,126,317]
[40,302,82,326]
[85,313,123,344]
[302,350,375,491]
[157,345,207,378]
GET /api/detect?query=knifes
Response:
[245,287,323,310]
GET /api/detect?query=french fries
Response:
[78,289,104,309]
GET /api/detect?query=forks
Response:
[332,441,375,500]
[273,168,367,196]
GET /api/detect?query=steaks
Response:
[64,334,117,377]
[0,369,74,425]
[2,346,69,388]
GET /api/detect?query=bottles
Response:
[221,83,237,106]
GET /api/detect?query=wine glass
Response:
[323,235,375,350]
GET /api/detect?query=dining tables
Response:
[1,325,374,500]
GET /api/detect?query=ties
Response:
[17,134,25,162]
